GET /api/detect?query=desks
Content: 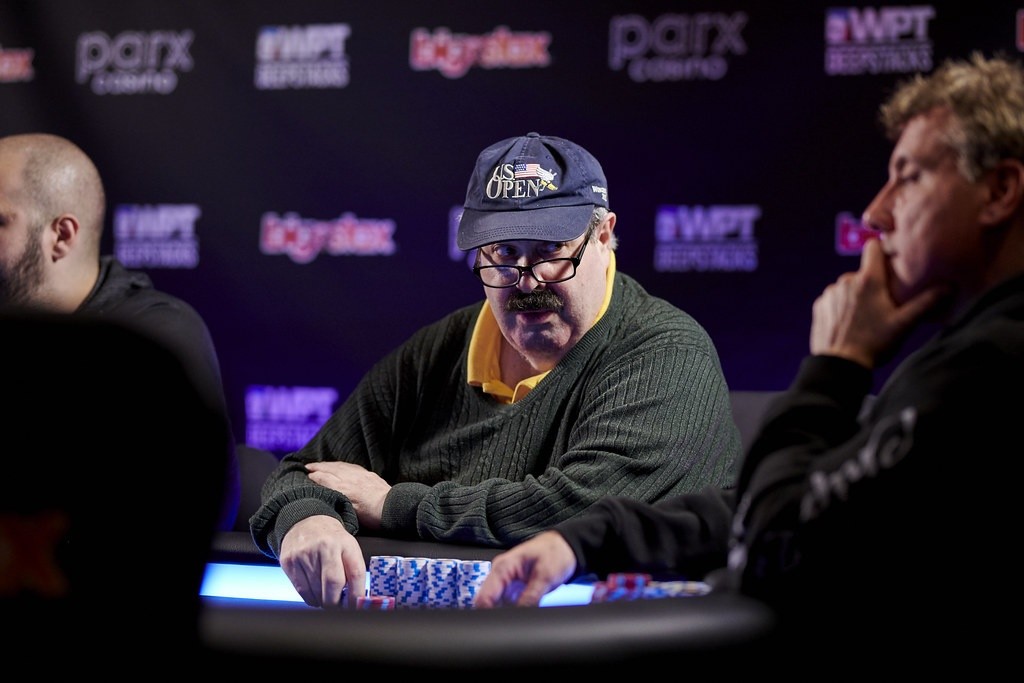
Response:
[203,532,783,683]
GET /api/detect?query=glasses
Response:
[471,213,599,288]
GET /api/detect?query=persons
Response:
[476,53,1024,683]
[0,133,241,531]
[249,133,746,610]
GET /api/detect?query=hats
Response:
[456,133,608,251]
